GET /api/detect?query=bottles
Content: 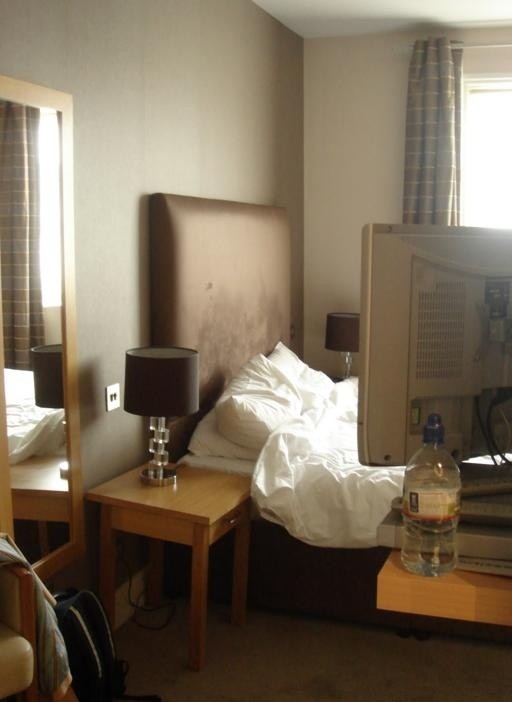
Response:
[403,415,463,578]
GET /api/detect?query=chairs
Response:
[0,563,163,702]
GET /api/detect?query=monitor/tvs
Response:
[358,222,512,465]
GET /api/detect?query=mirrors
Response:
[0,74,89,586]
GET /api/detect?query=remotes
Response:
[391,496,512,525]
[461,476,512,496]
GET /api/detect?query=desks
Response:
[84,460,258,672]
[375,550,512,627]
[8,452,71,559]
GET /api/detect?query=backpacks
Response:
[46,586,129,701]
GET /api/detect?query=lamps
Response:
[124,345,200,486]
[326,313,360,379]
[29,344,69,479]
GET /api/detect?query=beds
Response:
[149,192,511,641]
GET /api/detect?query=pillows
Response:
[187,340,337,461]
[216,352,302,449]
